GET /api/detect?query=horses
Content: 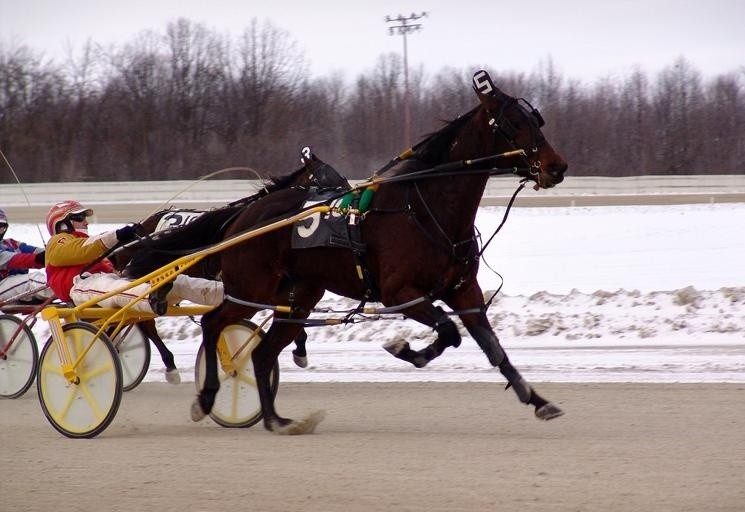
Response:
[107,150,352,387]
[123,69,567,434]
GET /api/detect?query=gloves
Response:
[116,226,131,241]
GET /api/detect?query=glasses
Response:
[69,212,86,222]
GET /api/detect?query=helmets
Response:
[46,199,93,237]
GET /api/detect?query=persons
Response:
[0,208,63,305]
[44,199,226,316]
